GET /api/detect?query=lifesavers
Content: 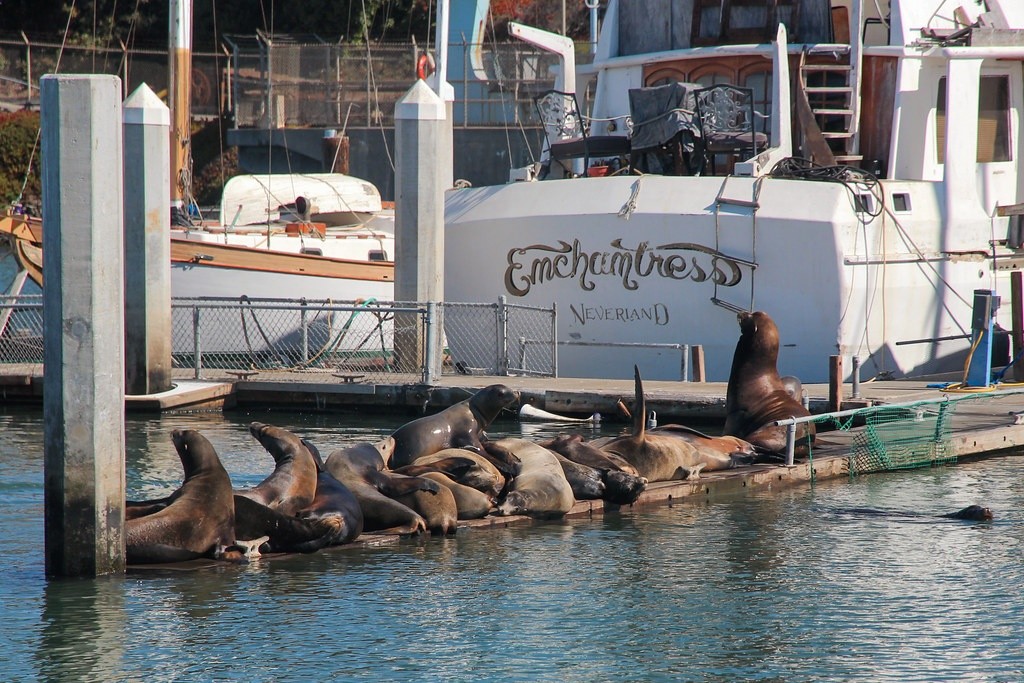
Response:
[417,52,436,81]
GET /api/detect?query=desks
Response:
[625,80,715,170]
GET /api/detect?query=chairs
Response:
[532,90,631,178]
[694,83,769,176]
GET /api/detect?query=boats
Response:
[446,0,1024,385]
[0,0,558,358]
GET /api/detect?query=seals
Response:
[124,364,756,571]
[721,312,817,461]
